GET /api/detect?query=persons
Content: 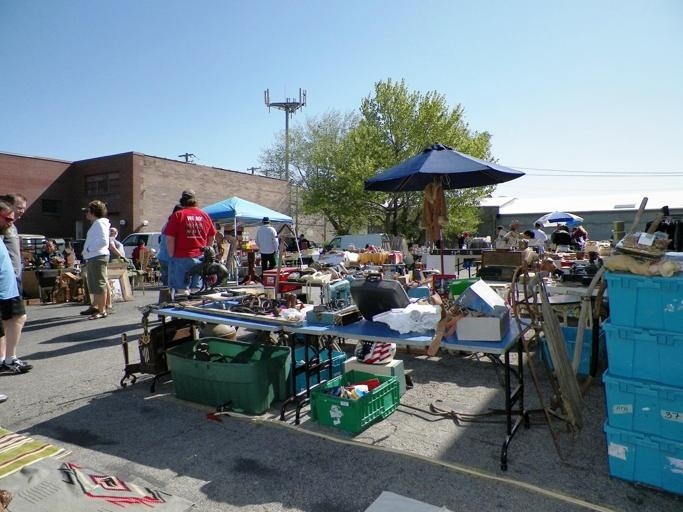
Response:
[0,202,33,374]
[132,238,149,271]
[157,204,184,322]
[163,189,216,321]
[254,217,280,278]
[458,223,588,253]
[280,234,311,251]
[80,207,110,315]
[34,240,65,302]
[0,193,27,364]
[109,227,126,260]
[82,200,111,320]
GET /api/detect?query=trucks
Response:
[326,233,391,254]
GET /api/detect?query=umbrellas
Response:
[363,141,525,253]
[533,211,585,230]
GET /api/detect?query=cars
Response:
[18,234,86,254]
[117,232,173,258]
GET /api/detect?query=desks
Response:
[282,318,533,470]
[151,301,306,421]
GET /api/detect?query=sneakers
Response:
[105,305,118,315]
[1,359,33,375]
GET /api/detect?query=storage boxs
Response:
[166,337,291,415]
[289,345,346,394]
[541,327,594,378]
[600,269,683,497]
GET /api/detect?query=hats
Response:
[181,188,196,200]
[261,217,270,223]
[80,199,107,216]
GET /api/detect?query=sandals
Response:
[79,306,100,315]
[87,312,107,320]
